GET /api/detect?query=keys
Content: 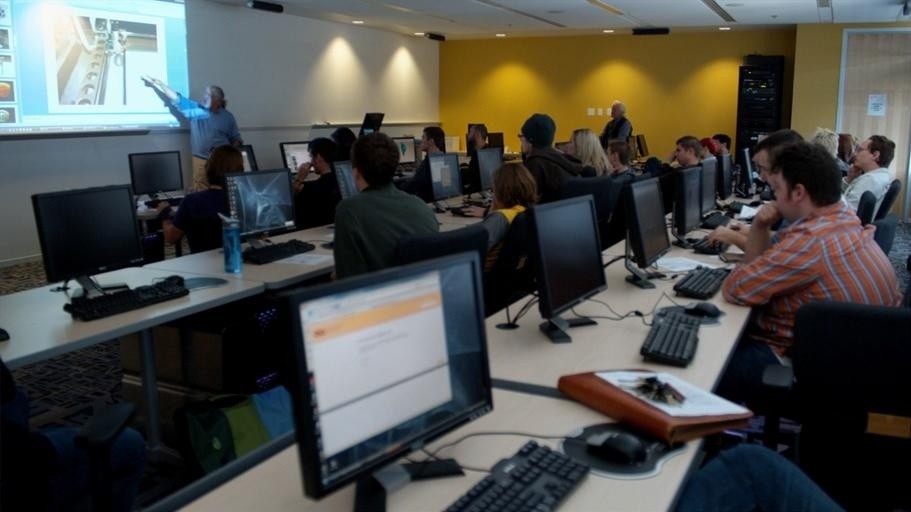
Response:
[623,377,685,406]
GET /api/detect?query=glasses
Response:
[517,130,524,141]
[855,143,867,154]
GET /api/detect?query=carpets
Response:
[887,223,911,303]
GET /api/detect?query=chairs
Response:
[858,193,876,224]
[794,303,910,511]
[872,178,901,257]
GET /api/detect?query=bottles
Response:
[223,220,241,274]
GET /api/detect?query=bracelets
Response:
[296,179,303,184]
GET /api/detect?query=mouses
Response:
[685,302,719,318]
[749,200,764,207]
[165,275,185,286]
[588,430,646,466]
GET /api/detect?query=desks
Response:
[666,191,760,223]
[430,188,493,225]
[146,379,705,512]
[142,223,466,408]
[603,217,744,263]
[1,266,267,461]
[485,255,755,391]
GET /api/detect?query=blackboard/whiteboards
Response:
[185,2,443,129]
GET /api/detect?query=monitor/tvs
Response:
[233,145,257,173]
[333,160,359,200]
[735,148,753,199]
[476,148,502,190]
[715,154,732,211]
[627,136,636,165]
[392,137,417,171]
[698,158,716,219]
[357,111,384,136]
[555,142,572,151]
[32,183,147,319]
[427,153,462,202]
[671,166,702,248]
[635,135,649,158]
[279,250,493,501]
[129,151,184,199]
[280,141,316,175]
[225,168,297,249]
[524,194,607,341]
[624,177,670,288]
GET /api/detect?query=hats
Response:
[521,112,556,145]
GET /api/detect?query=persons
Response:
[465,122,493,194]
[333,133,438,275]
[332,126,354,152]
[596,102,632,150]
[402,128,446,197]
[714,132,733,162]
[158,146,241,252]
[605,140,638,181]
[462,113,586,215]
[703,125,856,256]
[839,136,896,217]
[150,76,242,188]
[464,159,541,266]
[724,140,902,377]
[289,137,343,229]
[696,137,715,162]
[808,126,847,174]
[677,445,846,511]
[570,128,612,174]
[659,136,701,197]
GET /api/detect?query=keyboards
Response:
[700,212,725,229]
[674,266,731,300]
[445,441,591,512]
[78,280,189,320]
[693,235,729,254]
[145,198,187,208]
[639,308,701,369]
[728,200,748,213]
[242,239,316,265]
